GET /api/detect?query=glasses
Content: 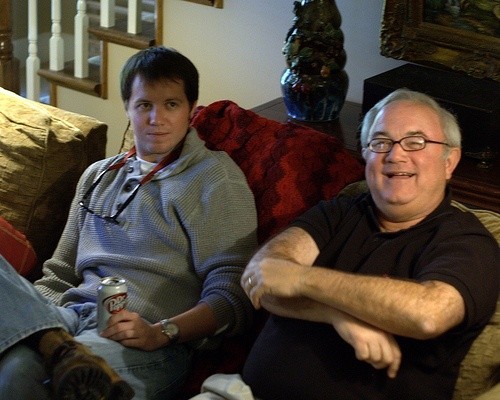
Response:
[78,166,142,225]
[365,136,451,153]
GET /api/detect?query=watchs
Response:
[159,317,181,345]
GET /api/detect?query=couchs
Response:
[188,100,500,400]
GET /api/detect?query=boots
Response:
[35,327,136,400]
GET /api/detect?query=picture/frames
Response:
[379,0,500,81]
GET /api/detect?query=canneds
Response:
[97,276,128,337]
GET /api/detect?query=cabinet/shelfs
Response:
[357,64,500,188]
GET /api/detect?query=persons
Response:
[0,44,257,400]
[187,87,499,400]
